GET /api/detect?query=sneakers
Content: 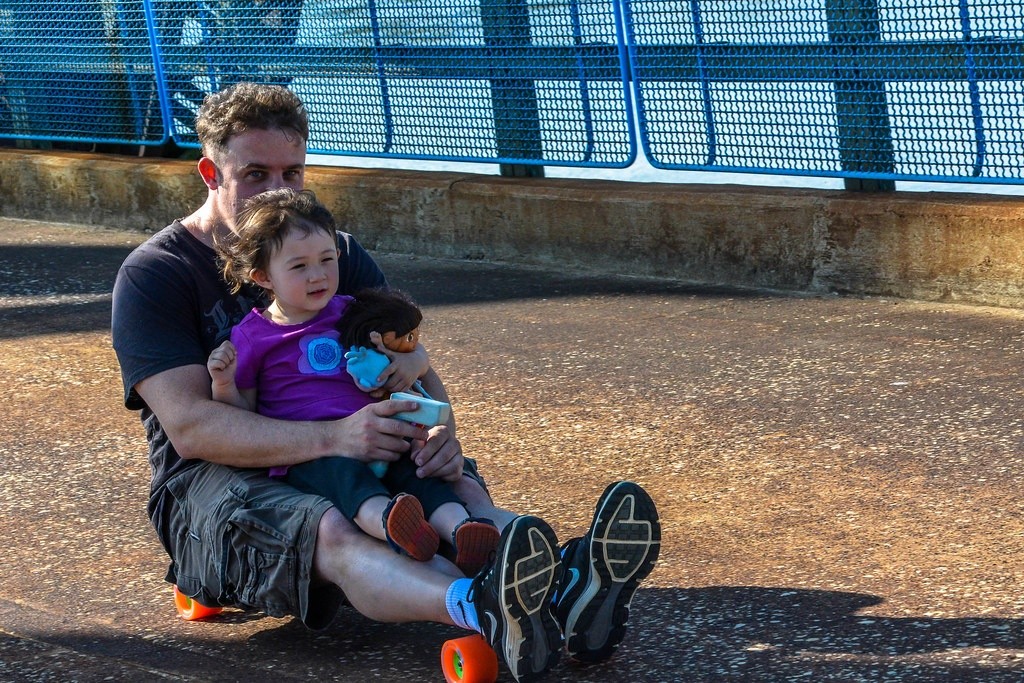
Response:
[549,481,661,664]
[467,515,562,683]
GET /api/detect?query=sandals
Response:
[382,493,440,562]
[451,517,500,578]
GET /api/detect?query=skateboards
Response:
[171,581,625,683]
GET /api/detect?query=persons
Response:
[339,288,426,476]
[207,188,500,578]
[111,84,663,683]
